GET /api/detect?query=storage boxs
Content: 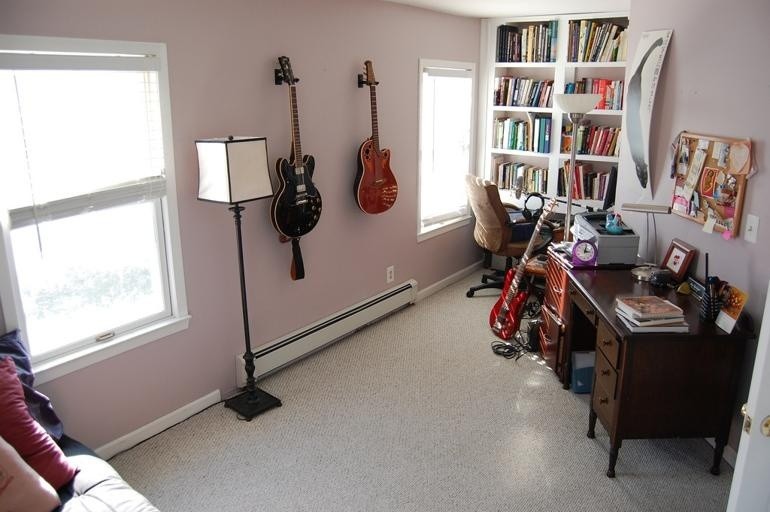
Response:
[571,350,597,394]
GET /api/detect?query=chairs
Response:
[466,174,555,298]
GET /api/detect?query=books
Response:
[614,295,691,336]
[488,21,631,203]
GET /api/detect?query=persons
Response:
[705,169,714,190]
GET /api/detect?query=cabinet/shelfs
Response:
[476,11,630,216]
[539,246,757,478]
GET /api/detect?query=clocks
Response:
[572,240,598,267]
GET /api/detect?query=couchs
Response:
[0,328,161,512]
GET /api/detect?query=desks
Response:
[554,229,573,242]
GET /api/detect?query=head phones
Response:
[522,192,544,219]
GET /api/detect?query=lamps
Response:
[553,94,604,243]
[194,135,282,422]
[621,203,671,282]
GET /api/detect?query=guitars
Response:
[353,60,398,215]
[490,199,557,339]
[277,56,322,238]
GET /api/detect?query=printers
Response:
[570,211,640,269]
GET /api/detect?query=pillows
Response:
[0,329,80,512]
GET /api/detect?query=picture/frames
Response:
[661,240,696,280]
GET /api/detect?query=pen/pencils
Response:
[709,276,729,319]
